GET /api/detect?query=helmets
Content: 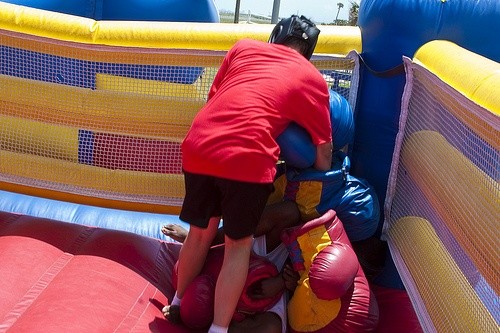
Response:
[269,11,320,62]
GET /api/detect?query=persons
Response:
[161,14,334,333]
[162,200,302,332]
